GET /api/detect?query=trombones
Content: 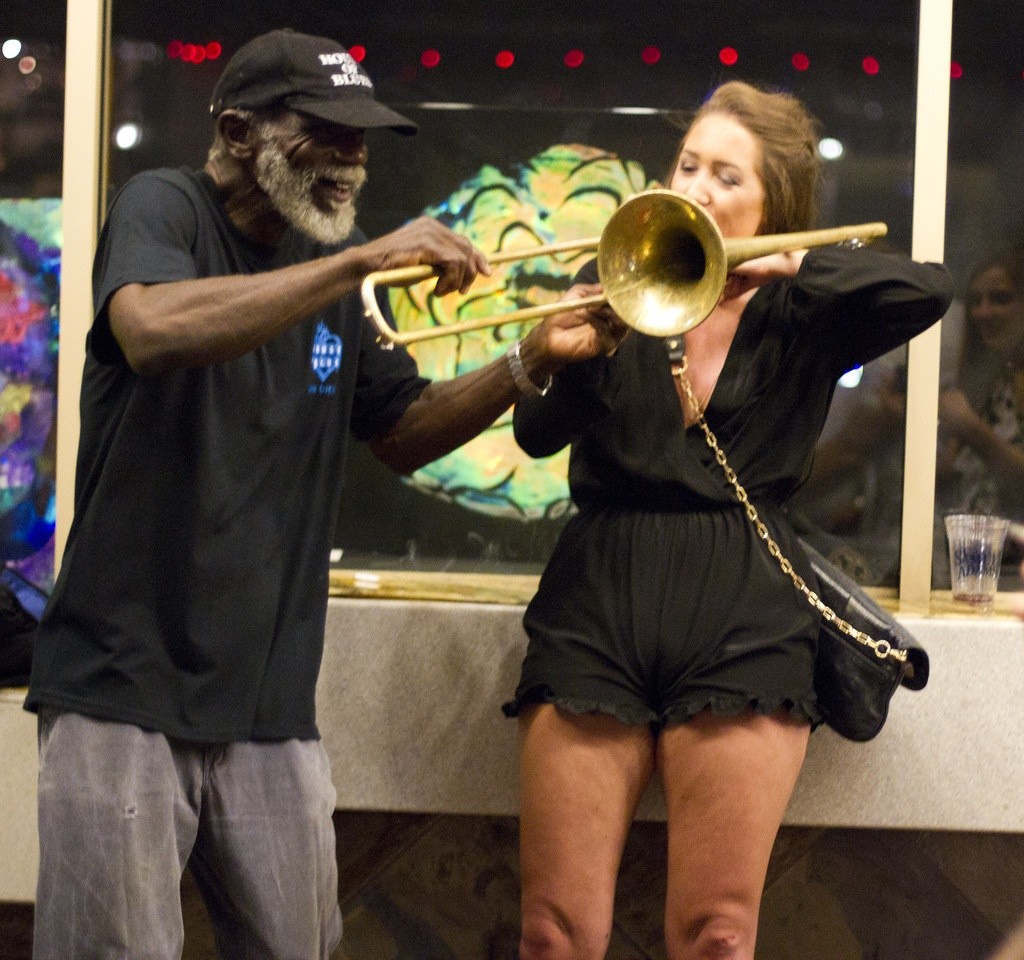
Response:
[356,185,892,354]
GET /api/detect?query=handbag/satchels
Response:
[796,538,929,741]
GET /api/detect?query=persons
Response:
[24,30,633,960]
[790,255,1024,593]
[502,82,953,960]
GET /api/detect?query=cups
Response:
[945,514,1010,604]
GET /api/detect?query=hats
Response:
[209,28,419,137]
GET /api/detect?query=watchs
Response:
[507,339,554,398]
